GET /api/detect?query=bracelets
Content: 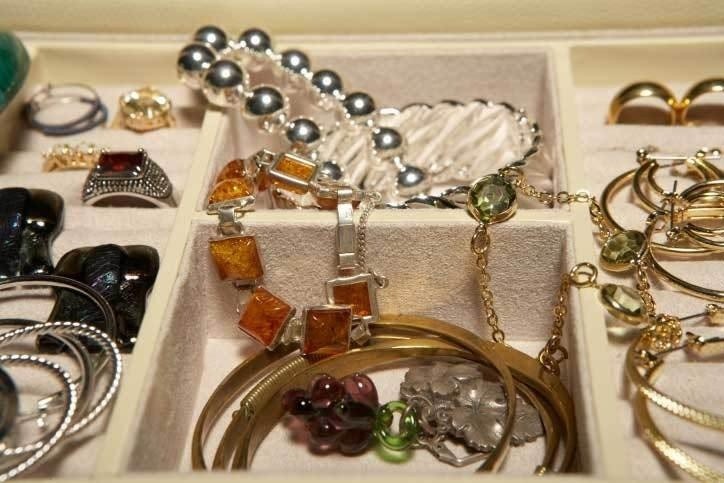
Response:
[0,270,123,482]
[205,147,382,360]
[187,308,576,479]
[601,148,724,482]
[305,98,558,209]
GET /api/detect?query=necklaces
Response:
[461,169,655,368]
[176,26,432,203]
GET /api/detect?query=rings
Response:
[37,140,108,174]
[111,84,176,128]
[609,79,675,123]
[83,151,181,209]
[676,73,723,126]
